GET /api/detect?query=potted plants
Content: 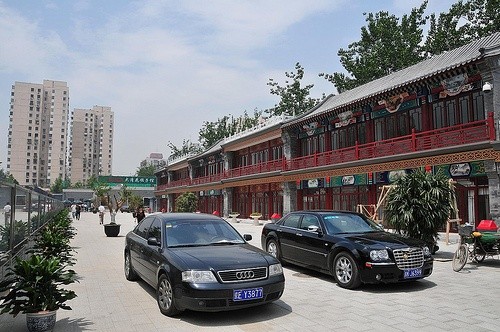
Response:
[385,165,456,256]
[93,182,132,237]
[0,208,78,332]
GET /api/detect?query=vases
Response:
[250,215,262,225]
[230,213,240,223]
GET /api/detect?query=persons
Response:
[137,206,145,223]
[71,203,105,224]
[2,201,11,227]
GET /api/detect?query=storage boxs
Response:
[476,219,498,233]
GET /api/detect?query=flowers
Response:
[251,211,260,215]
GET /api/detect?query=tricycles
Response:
[452,220,500,272]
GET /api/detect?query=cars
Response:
[124,212,286,318]
[261,209,434,291]
